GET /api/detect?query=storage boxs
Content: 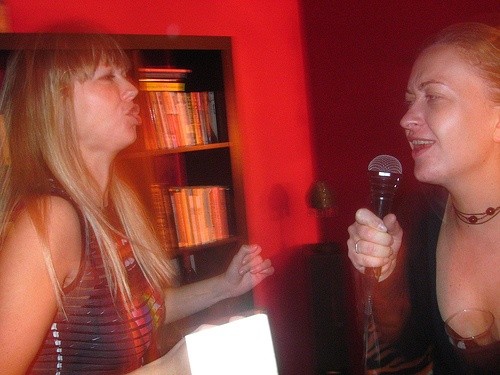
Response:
[138,72,185,91]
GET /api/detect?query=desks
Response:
[309,243,351,375]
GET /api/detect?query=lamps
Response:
[306,181,337,252]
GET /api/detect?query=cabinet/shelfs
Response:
[0,32,255,343]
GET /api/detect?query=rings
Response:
[355,240,360,254]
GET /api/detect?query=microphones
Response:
[367,156,403,309]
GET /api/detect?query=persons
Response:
[0,21,276,375]
[347,22,500,375]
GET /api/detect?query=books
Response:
[136,67,220,151]
[142,183,229,250]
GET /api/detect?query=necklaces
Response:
[451,199,500,225]
